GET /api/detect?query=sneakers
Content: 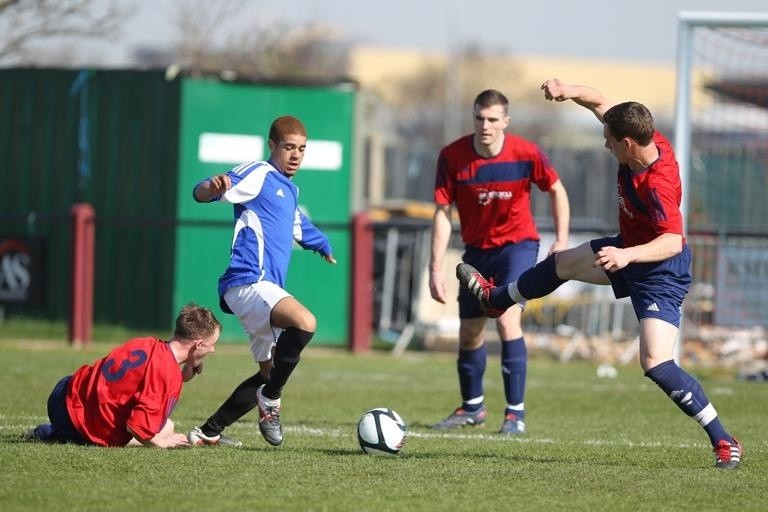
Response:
[26,422,52,442]
[187,425,244,448]
[712,436,744,469]
[499,408,526,434]
[255,383,284,446]
[429,405,488,430]
[456,262,507,320]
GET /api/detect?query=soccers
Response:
[357,406,408,456]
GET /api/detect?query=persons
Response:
[429,90,570,436]
[25,304,223,449]
[457,79,745,471]
[187,116,337,448]
